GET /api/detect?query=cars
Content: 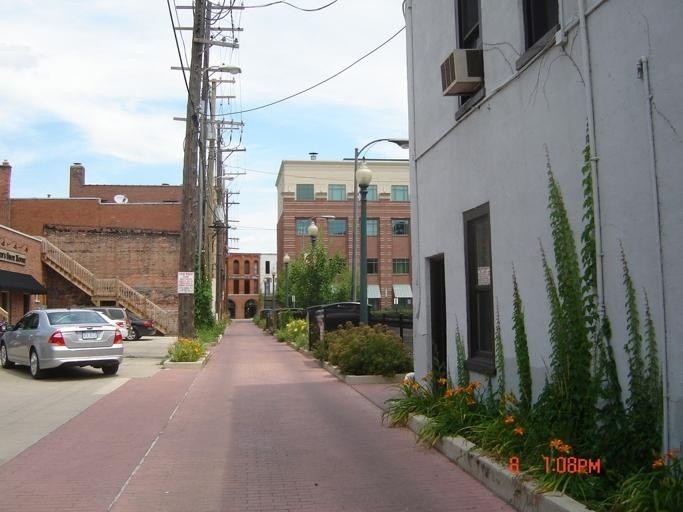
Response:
[93,307,157,341]
[1,308,123,379]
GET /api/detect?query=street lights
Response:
[351,139,408,326]
[262,278,267,309]
[307,220,319,249]
[283,254,292,307]
[272,266,276,312]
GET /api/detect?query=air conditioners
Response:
[439,49,483,97]
